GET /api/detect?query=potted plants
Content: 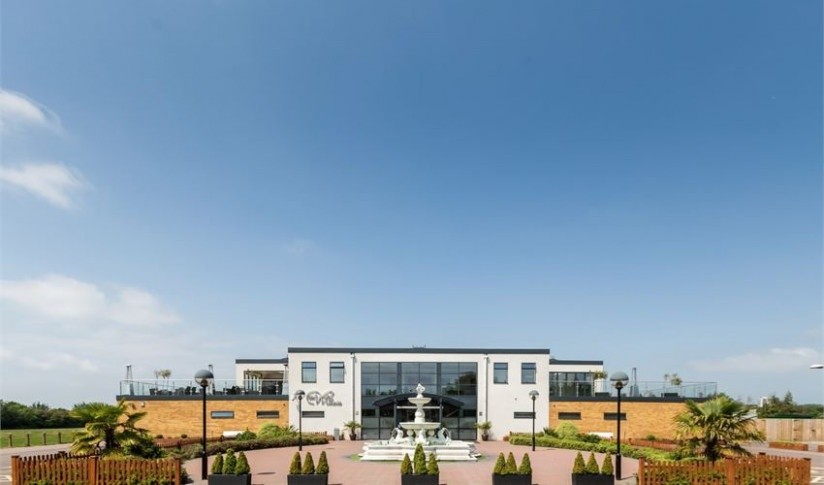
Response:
[492,451,533,485]
[287,451,329,485]
[474,420,492,441]
[400,442,440,485]
[208,447,252,485]
[571,451,615,485]
[344,420,364,440]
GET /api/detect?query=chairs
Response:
[149,385,276,395]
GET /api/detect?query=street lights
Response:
[195,370,215,479]
[529,390,539,450]
[610,372,629,481]
[294,390,305,451]
